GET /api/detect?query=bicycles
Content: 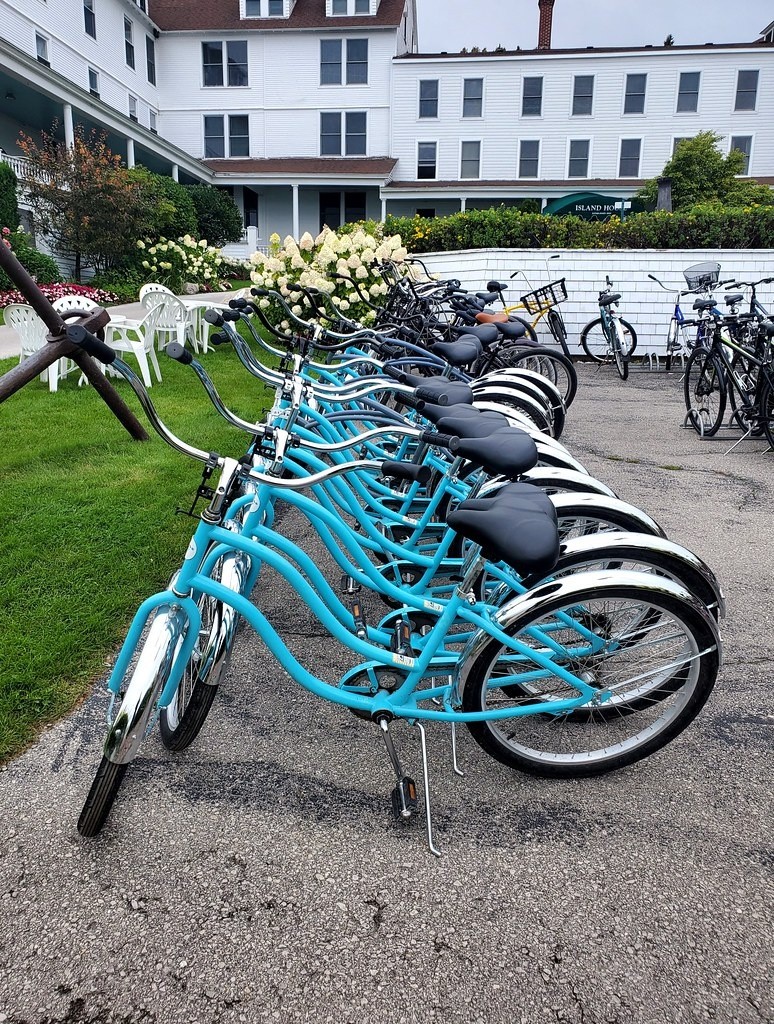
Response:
[648,274,774,453]
[62,252,726,856]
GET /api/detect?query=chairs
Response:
[54,296,100,377]
[103,302,165,388]
[139,284,179,342]
[141,292,199,354]
[4,303,90,394]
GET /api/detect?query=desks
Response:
[64,314,125,377]
[173,299,238,355]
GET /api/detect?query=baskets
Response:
[519,278,568,315]
[682,262,722,295]
